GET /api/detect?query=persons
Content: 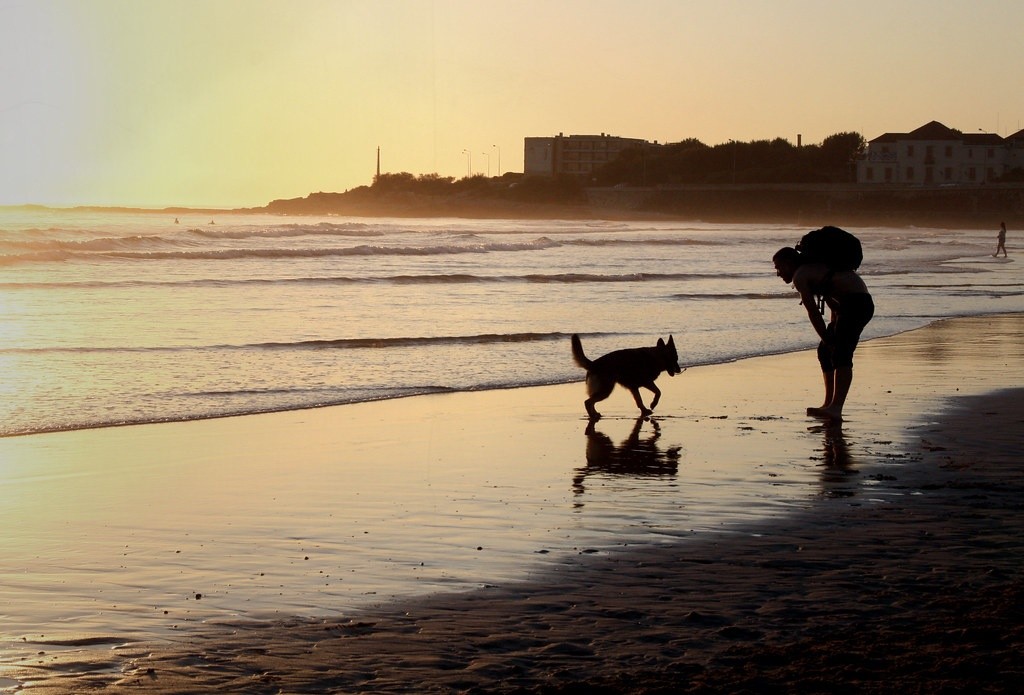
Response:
[991,222,1007,258]
[773,246,874,419]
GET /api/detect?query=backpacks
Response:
[794,225,863,315]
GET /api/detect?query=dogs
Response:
[570,334,682,418]
